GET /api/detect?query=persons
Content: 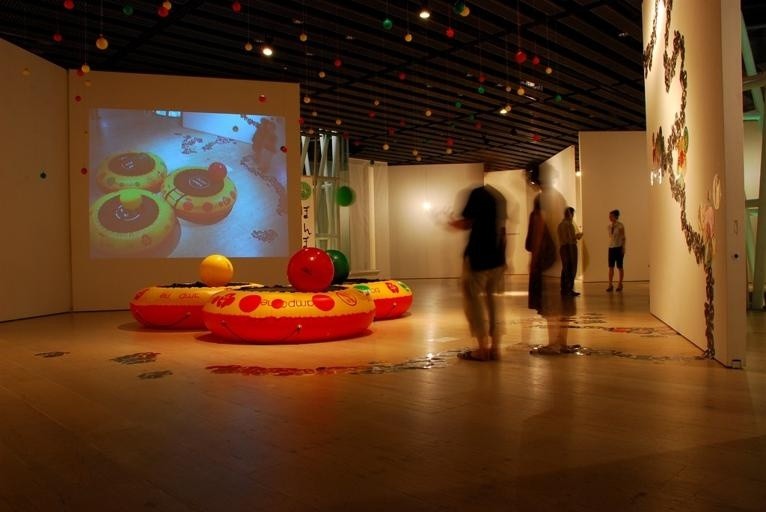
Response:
[521,158,582,355]
[256,121,277,171]
[607,210,626,291]
[557,208,584,297]
[448,183,520,362]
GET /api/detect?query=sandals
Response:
[458,351,497,361]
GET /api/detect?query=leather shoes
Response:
[560,290,580,296]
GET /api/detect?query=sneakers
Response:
[606,285,623,291]
[530,344,567,355]
